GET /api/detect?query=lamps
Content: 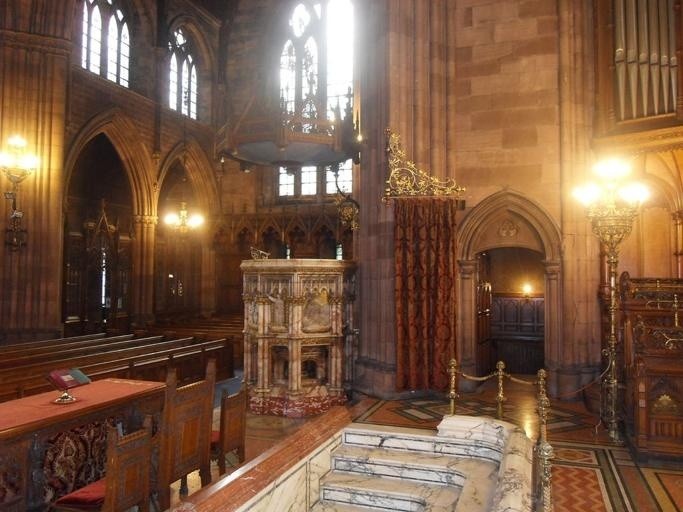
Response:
[213,0,368,177]
[584,189,642,445]
[0,133,41,251]
[163,89,205,234]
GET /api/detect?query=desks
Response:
[0,378,169,512]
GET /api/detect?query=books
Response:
[49,367,91,389]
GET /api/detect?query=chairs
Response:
[51,359,249,512]
